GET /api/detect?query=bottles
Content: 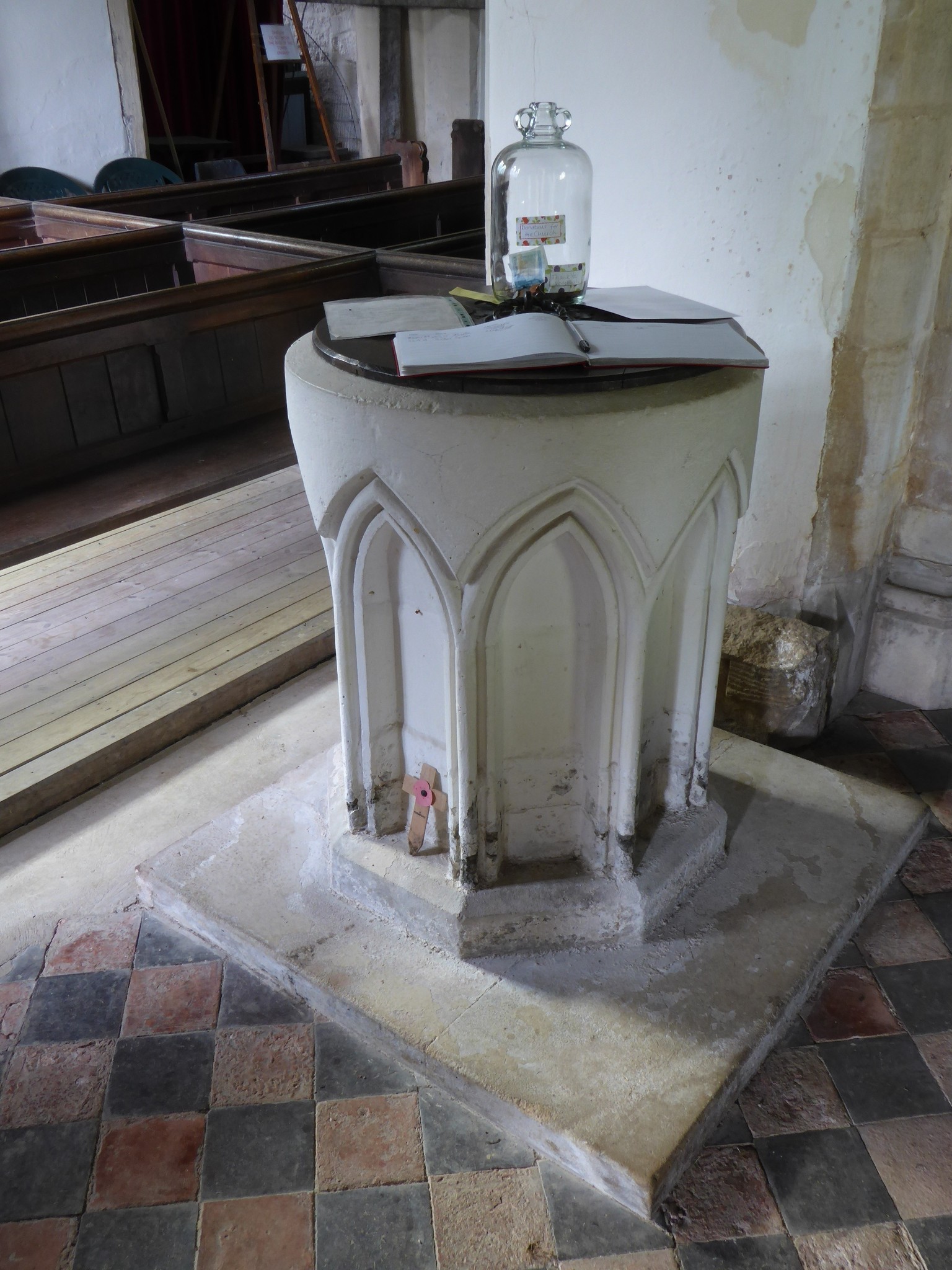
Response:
[487,100,596,301]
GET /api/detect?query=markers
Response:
[564,318,590,352]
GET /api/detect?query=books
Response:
[392,312,769,379]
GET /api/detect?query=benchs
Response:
[0,153,486,580]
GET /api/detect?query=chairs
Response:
[91,157,185,195]
[195,159,247,183]
[0,166,89,202]
